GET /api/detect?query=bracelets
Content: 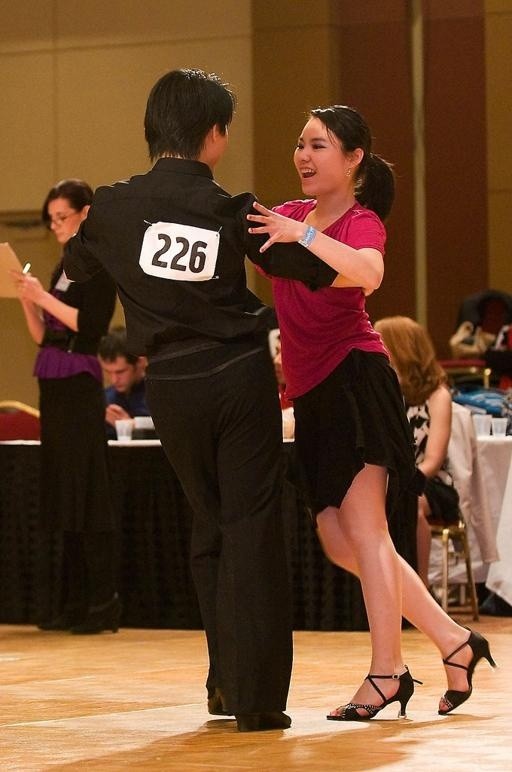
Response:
[298,225,316,249]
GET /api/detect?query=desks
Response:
[0,437,417,634]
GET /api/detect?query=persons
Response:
[97,325,153,439]
[63,67,380,731]
[239,104,497,725]
[269,333,298,409]
[8,178,125,634]
[369,315,460,595]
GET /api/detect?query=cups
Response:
[115,417,155,441]
[471,415,508,438]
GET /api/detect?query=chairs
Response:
[0,401,42,441]
[426,402,479,624]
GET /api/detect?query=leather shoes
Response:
[208,686,291,732]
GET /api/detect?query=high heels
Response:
[438,625,499,714]
[36,601,122,634]
[327,665,414,721]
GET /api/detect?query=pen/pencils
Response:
[23,263,31,273]
